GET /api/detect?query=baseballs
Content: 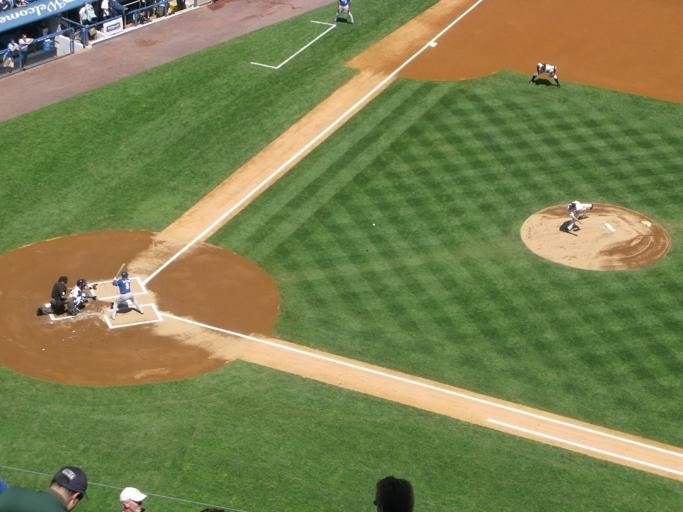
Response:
[372,223,376,227]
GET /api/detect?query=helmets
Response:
[59,276,67,284]
[121,272,128,279]
[77,279,86,288]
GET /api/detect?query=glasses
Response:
[133,501,142,505]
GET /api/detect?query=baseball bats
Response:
[115,264,125,278]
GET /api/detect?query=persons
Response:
[0,1,188,72]
[69,278,88,312]
[112,272,144,319]
[119,486,147,512]
[334,0,354,25]
[375,475,415,512]
[36,275,77,317]
[565,200,594,231]
[529,62,560,88]
[0,465,89,512]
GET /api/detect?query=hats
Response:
[120,487,147,502]
[53,467,89,500]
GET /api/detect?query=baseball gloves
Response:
[81,292,88,301]
